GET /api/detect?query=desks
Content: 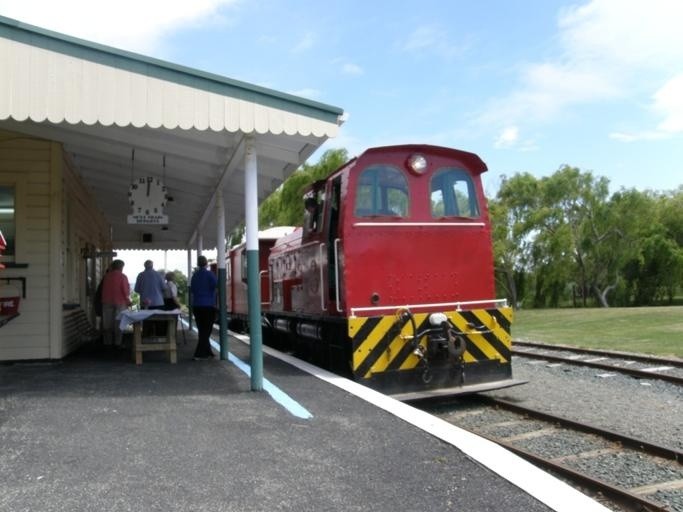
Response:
[123,309,182,366]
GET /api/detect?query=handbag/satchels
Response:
[163,297,178,311]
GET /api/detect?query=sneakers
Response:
[192,354,216,361]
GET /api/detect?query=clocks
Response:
[127,175,170,217]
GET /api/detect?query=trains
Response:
[209,141,526,402]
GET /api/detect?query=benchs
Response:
[59,302,116,362]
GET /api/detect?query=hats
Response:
[197,255,207,266]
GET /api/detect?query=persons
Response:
[133,259,167,338]
[92,278,102,331]
[162,273,180,310]
[101,261,131,350]
[303,197,318,215]
[188,255,219,361]
